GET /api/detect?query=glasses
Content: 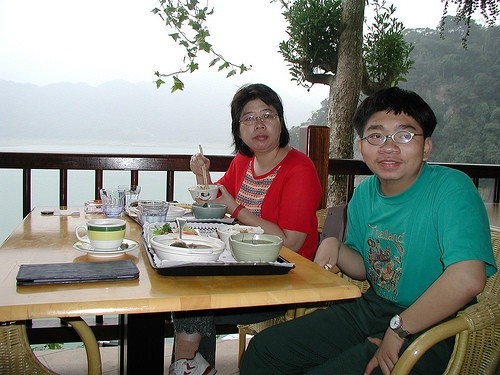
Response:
[239,112,281,124]
[358,130,425,145]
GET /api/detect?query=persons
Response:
[169,84,322,375]
[242,89,498,375]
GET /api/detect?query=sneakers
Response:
[169,350,217,375]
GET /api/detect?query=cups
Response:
[75,219,127,251]
[117,185,141,205]
[99,188,126,216]
[137,200,169,226]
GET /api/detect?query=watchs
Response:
[390,315,411,338]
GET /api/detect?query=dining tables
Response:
[0,204,362,375]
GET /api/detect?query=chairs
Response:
[295,225,500,375]
[0,317,102,375]
[237,207,346,368]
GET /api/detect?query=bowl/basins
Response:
[229,232,283,262]
[191,204,227,220]
[216,224,265,250]
[187,184,219,203]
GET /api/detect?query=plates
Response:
[72,238,139,258]
[165,206,186,220]
[151,234,226,263]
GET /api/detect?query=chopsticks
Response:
[198,143,208,189]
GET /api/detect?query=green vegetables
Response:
[153,223,173,235]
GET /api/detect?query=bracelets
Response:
[231,205,244,221]
